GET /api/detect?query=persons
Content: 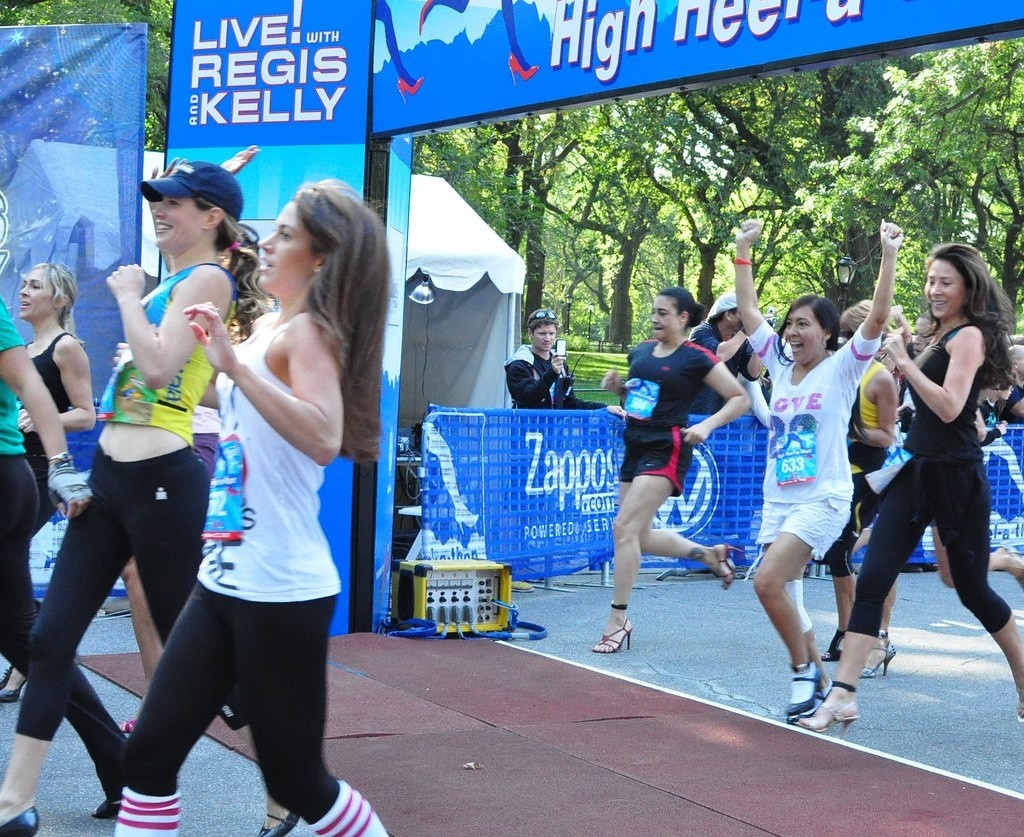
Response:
[112,177,390,837]
[0,144,299,837]
[811,300,1024,676]
[592,287,751,652]
[504,308,627,592]
[734,219,904,726]
[794,244,1024,734]
[0,144,275,817]
[687,291,775,419]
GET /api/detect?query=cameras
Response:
[555,338,566,358]
[767,307,775,316]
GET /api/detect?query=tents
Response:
[0,140,526,533]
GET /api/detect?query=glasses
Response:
[529,312,557,322]
[846,331,854,340]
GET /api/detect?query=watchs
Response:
[48,452,73,462]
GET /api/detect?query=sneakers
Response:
[787,682,832,725]
[785,664,821,716]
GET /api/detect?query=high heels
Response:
[1016,687,1024,722]
[0,676,28,702]
[860,631,896,678]
[996,543,1024,590]
[795,681,859,739]
[592,617,633,652]
[713,543,744,590]
[818,629,846,662]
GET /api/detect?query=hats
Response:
[142,159,244,224]
[709,291,737,318]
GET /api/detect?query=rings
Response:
[24,423,27,426]
[216,308,221,313]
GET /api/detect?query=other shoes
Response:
[119,720,137,734]
[259,805,300,837]
[0,805,38,837]
[94,796,122,817]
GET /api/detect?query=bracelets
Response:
[734,258,753,265]
